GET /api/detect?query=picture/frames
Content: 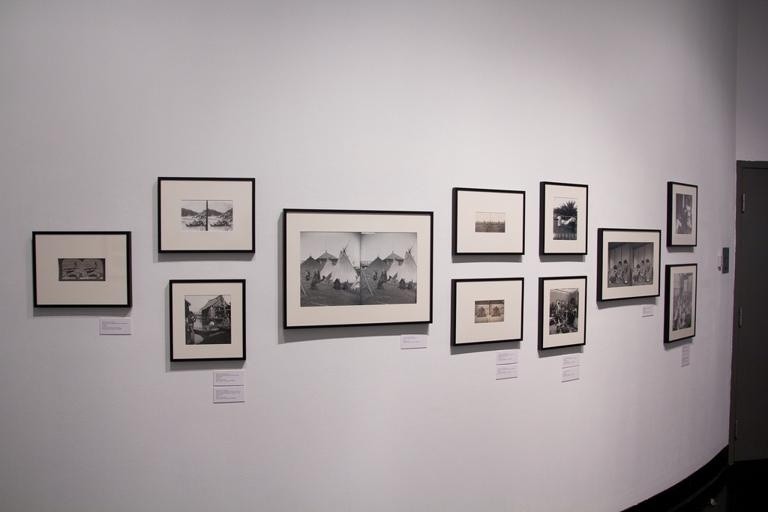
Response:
[450,184,528,257]
[279,207,436,330]
[666,181,699,247]
[30,229,133,310]
[663,263,698,343]
[155,176,257,364]
[596,226,661,302]
[538,180,589,256]
[449,276,524,344]
[538,275,588,351]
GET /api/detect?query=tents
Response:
[301,247,417,297]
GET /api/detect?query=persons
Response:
[608,258,653,285]
[675,195,693,235]
[548,300,578,334]
